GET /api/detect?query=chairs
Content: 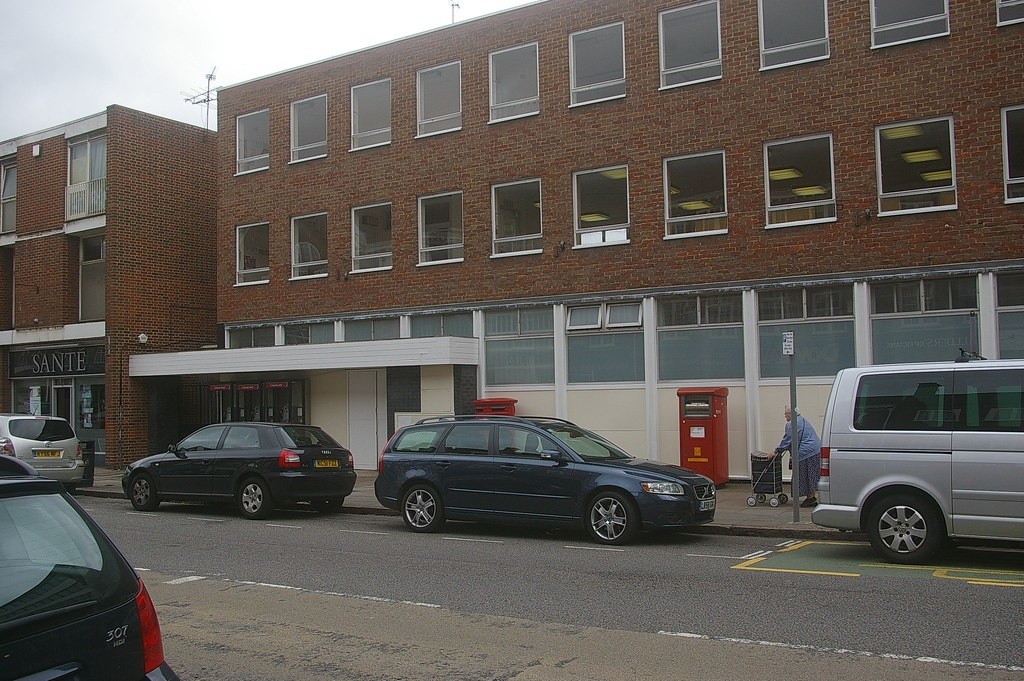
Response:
[524,434,540,453]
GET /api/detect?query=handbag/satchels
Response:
[789,458,793,470]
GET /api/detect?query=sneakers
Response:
[800,496,819,507]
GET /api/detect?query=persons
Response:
[768,405,821,507]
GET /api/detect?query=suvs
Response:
[120,421,357,520]
[371,416,717,545]
[0,451,182,681]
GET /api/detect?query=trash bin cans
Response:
[76,440,96,488]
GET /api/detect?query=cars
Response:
[808,346,1024,565]
[0,414,86,495]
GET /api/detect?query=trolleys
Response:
[746,452,789,507]
